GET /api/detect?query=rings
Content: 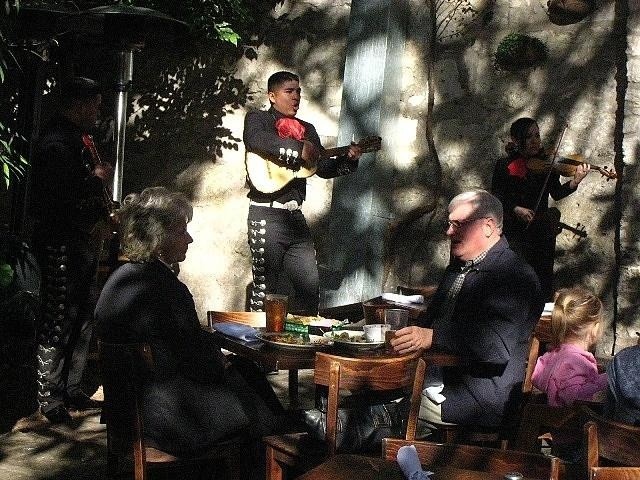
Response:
[410,341,412,347]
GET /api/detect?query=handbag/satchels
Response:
[294,379,408,455]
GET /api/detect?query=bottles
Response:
[504,472,523,480]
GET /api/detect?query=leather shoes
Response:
[40,386,104,426]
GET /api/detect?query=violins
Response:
[528,151,620,182]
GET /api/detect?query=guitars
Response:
[245,135,382,196]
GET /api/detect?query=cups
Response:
[265,293,289,332]
[364,301,409,351]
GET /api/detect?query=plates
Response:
[253,318,385,350]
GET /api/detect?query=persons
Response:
[390,189,545,442]
[597,332,640,466]
[243,71,362,317]
[93,185,285,480]
[492,117,590,303]
[531,290,609,462]
[26,76,115,424]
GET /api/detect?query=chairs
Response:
[207,295,640,479]
[95,340,241,480]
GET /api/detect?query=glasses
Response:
[443,216,492,228]
